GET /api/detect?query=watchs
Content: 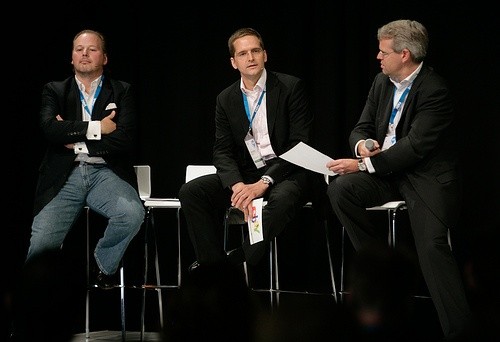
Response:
[357,159,367,171]
[262,177,273,186]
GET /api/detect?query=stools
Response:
[85,165,407,342]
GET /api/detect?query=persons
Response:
[26,29,144,303]
[178,27,309,274]
[328,18,469,342]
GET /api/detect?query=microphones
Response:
[365,139,376,152]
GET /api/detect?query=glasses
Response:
[379,49,396,58]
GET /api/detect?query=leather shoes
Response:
[96,270,114,288]
[188,249,242,276]
